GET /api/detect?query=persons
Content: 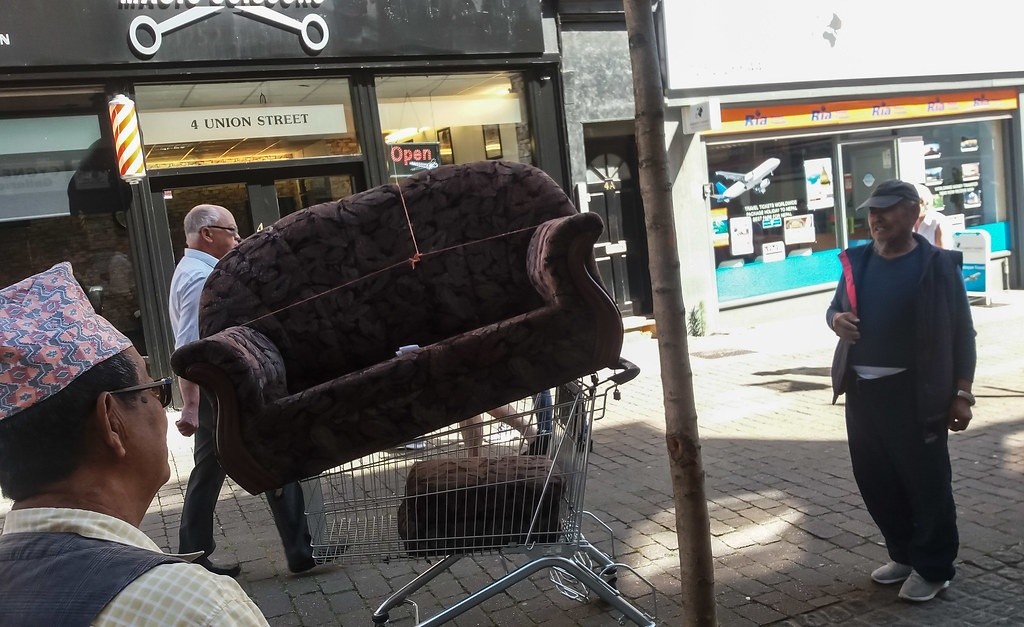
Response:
[0,261,272,627]
[108,235,145,356]
[459,377,594,458]
[168,203,352,578]
[826,181,977,602]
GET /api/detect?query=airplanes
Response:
[709,158,781,203]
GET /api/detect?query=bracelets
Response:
[953,389,975,405]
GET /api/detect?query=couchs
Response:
[168,158,625,501]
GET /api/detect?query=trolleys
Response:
[299,359,657,627]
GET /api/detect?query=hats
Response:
[0,262,133,421]
[855,180,919,212]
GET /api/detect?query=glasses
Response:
[199,225,238,236]
[93,376,172,408]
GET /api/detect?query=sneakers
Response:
[898,570,949,601]
[871,562,914,584]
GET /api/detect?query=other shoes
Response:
[395,435,427,449]
[206,564,241,578]
[521,429,552,455]
[289,538,350,574]
